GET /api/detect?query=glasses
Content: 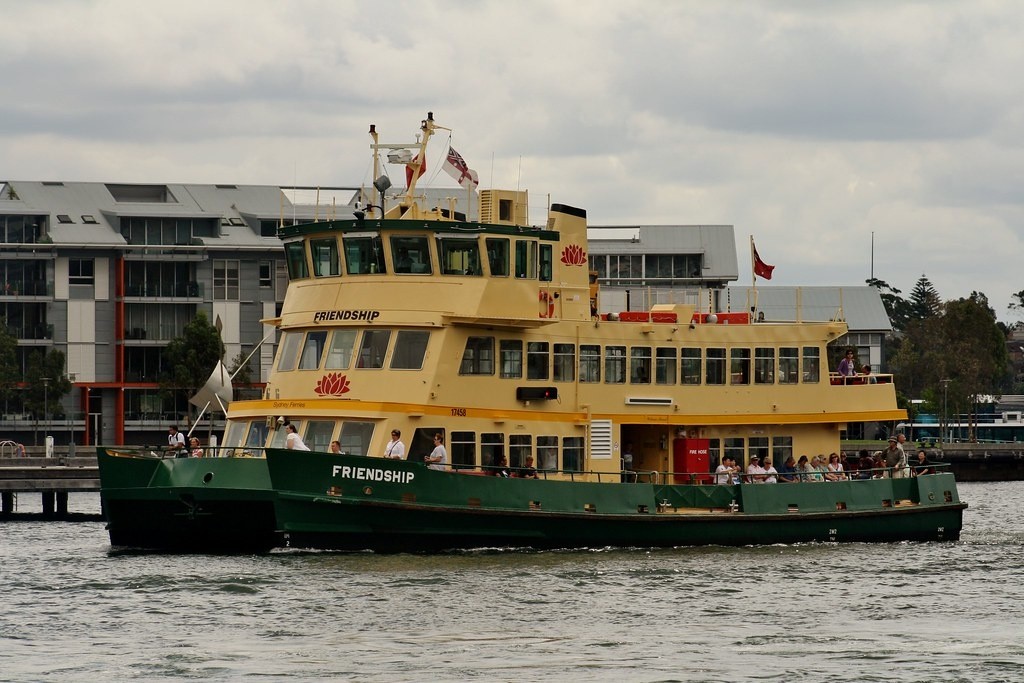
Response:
[751,459,758,462]
[830,456,836,460]
[847,352,853,356]
[766,462,772,465]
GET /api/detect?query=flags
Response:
[441,146,479,191]
[406,151,426,191]
[753,244,775,281]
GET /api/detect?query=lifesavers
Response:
[15,444,25,457]
[539,290,554,317]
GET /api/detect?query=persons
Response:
[746,454,771,484]
[494,455,512,478]
[331,441,342,453]
[519,455,540,479]
[167,424,189,458]
[861,364,878,383]
[795,455,812,483]
[885,434,911,478]
[424,433,446,471]
[806,453,847,481]
[842,449,887,480]
[713,455,744,485]
[384,429,405,459]
[779,456,799,483]
[837,349,859,385]
[913,450,931,476]
[285,424,311,451]
[762,456,779,484]
[636,367,649,383]
[621,442,634,483]
[189,437,203,458]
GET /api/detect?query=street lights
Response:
[939,379,953,443]
[66,372,79,457]
[40,377,53,446]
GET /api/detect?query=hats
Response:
[887,435,898,443]
[748,454,760,463]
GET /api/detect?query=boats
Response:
[96,112,969,554]
